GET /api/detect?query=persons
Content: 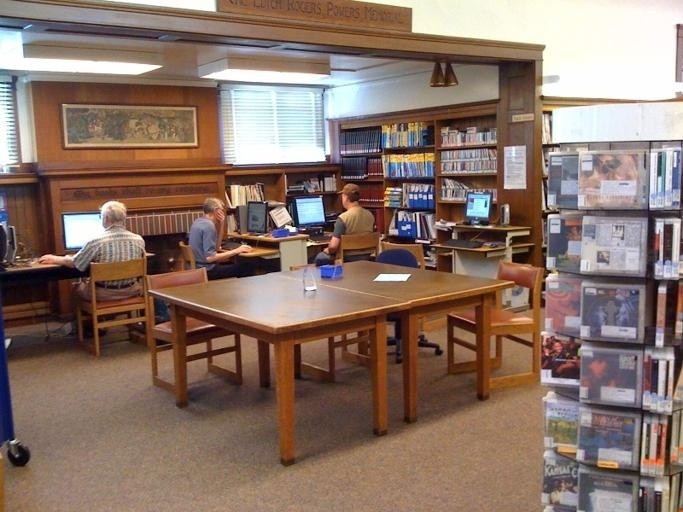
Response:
[187,197,255,277]
[38,201,146,339]
[313,183,375,266]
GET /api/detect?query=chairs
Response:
[174,241,205,275]
[376,240,429,271]
[141,266,246,396]
[444,257,547,404]
[334,229,383,266]
[73,258,156,364]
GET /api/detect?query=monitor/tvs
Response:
[465,192,493,225]
[61,211,109,254]
[289,194,328,228]
[248,201,270,235]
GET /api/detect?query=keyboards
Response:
[222,242,241,250]
[310,235,332,242]
[442,239,484,248]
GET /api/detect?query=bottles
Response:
[302,268,317,291]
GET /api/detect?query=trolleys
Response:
[1,223,31,466]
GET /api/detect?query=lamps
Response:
[428,59,458,87]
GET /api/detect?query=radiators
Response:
[125,209,205,237]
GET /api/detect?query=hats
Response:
[336,183,359,195]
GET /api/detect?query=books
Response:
[336,120,498,267]
[540,136,682,512]
[223,173,336,232]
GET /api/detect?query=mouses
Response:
[483,242,497,248]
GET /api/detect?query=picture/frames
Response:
[55,99,201,151]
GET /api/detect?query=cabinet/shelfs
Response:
[382,108,440,271]
[326,113,385,231]
[438,97,502,273]
[538,140,682,510]
[222,158,344,232]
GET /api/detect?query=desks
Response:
[0,247,157,346]
[277,260,516,426]
[431,219,535,272]
[218,229,313,273]
[295,223,339,266]
[145,273,410,468]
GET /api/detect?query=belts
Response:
[95,282,134,289]
